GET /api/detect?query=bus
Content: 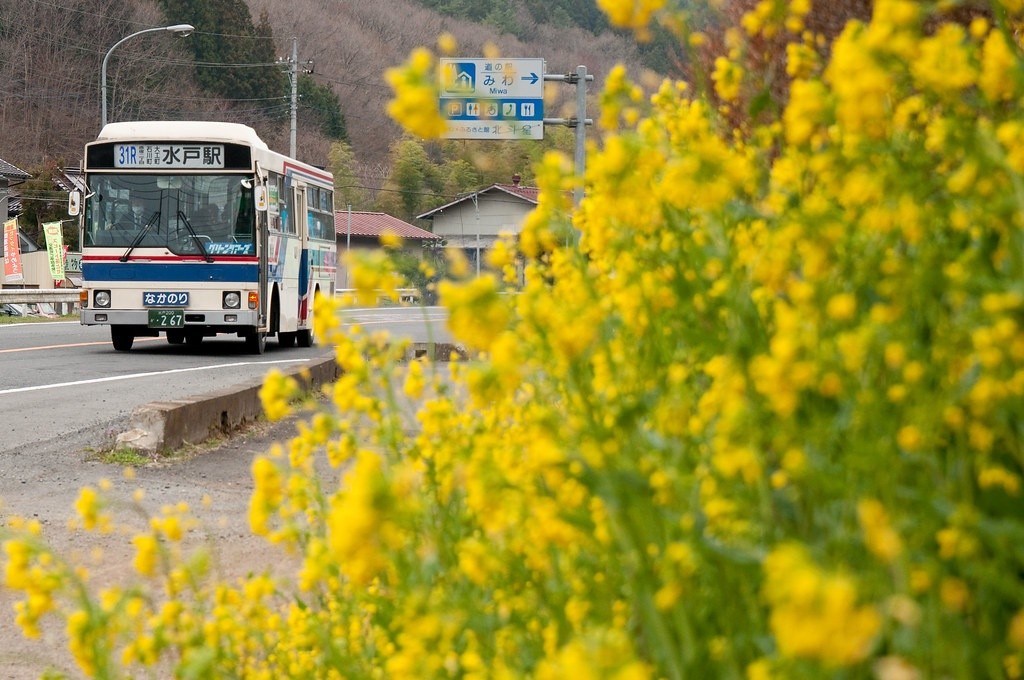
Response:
[68,121,337,355]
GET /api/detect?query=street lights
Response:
[101,24,195,130]
[346,202,352,289]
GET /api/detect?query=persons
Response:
[106,189,159,230]
[181,203,238,241]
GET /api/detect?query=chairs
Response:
[116,209,140,231]
